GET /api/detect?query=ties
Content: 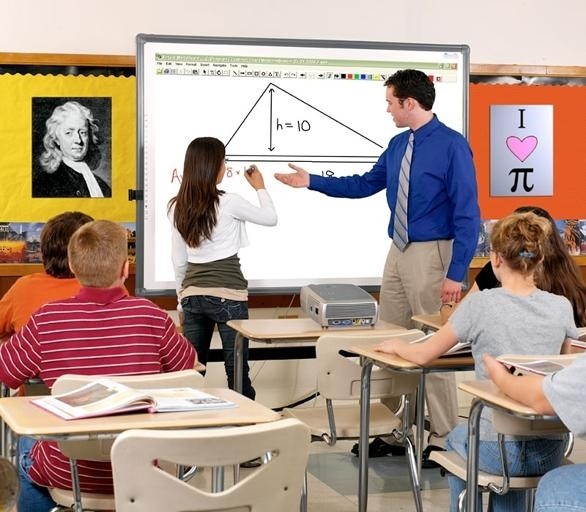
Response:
[392,133,415,251]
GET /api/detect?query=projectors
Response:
[300,283,379,332]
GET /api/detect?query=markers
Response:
[245,168,255,176]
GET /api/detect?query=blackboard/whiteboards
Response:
[135,34,469,298]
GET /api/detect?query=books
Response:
[495,353,583,377]
[29,379,239,421]
[410,332,472,357]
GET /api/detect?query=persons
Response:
[273,69,480,469]
[481,351,586,512]
[166,137,278,401]
[32,102,111,198]
[1,219,197,512]
[440,206,586,328]
[374,209,579,512]
[0,211,94,396]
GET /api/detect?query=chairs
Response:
[109,417,312,512]
[428,354,581,512]
[47,367,206,512]
[285,330,428,512]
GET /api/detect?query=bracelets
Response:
[440,304,452,312]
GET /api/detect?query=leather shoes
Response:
[416,445,447,468]
[351,438,405,457]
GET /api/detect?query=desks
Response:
[411,310,453,330]
[347,346,473,512]
[226,317,408,417]
[0,389,285,473]
[456,377,577,512]
